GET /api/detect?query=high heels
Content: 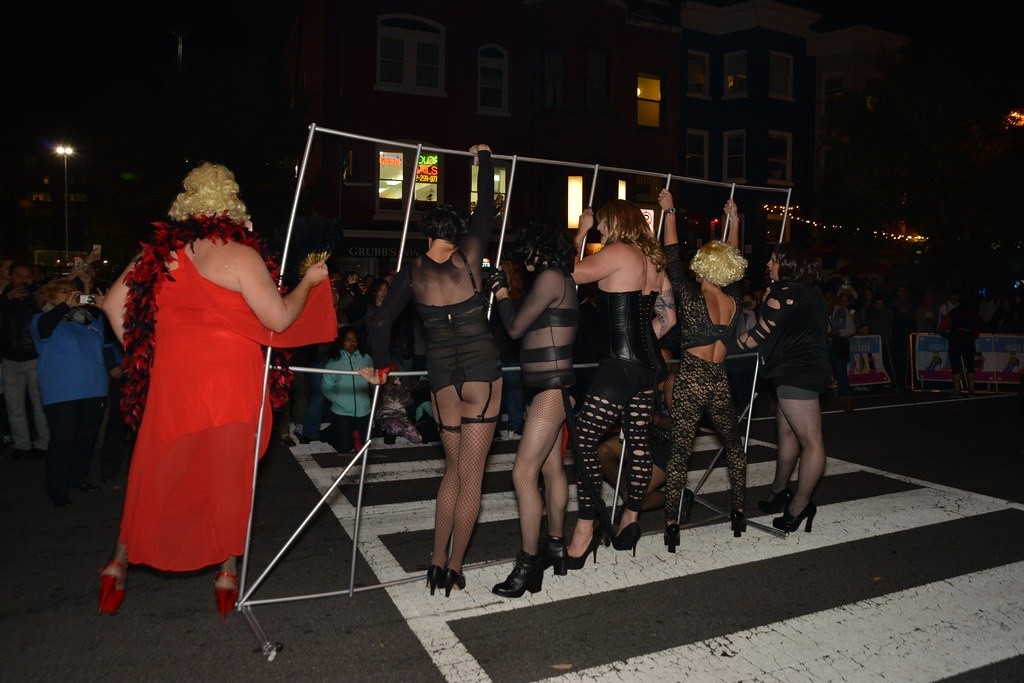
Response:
[98,560,129,615]
[493,551,543,598]
[445,568,465,598]
[215,571,240,620]
[664,523,680,553]
[612,522,641,557]
[566,528,601,569]
[731,511,747,537]
[426,565,445,596]
[541,537,567,575]
[773,501,816,532]
[763,487,793,513]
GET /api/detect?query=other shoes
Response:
[22,447,49,459]
[320,430,329,442]
[47,488,72,507]
[6,449,29,459]
[282,436,295,446]
[300,437,314,444]
[73,479,101,492]
[101,461,116,482]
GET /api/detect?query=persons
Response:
[98,164,338,614]
[0,248,142,493]
[825,269,1024,397]
[657,189,749,552]
[483,221,580,599]
[730,242,827,532]
[565,202,677,570]
[357,143,504,598]
[263,230,442,452]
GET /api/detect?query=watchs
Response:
[665,208,675,215]
